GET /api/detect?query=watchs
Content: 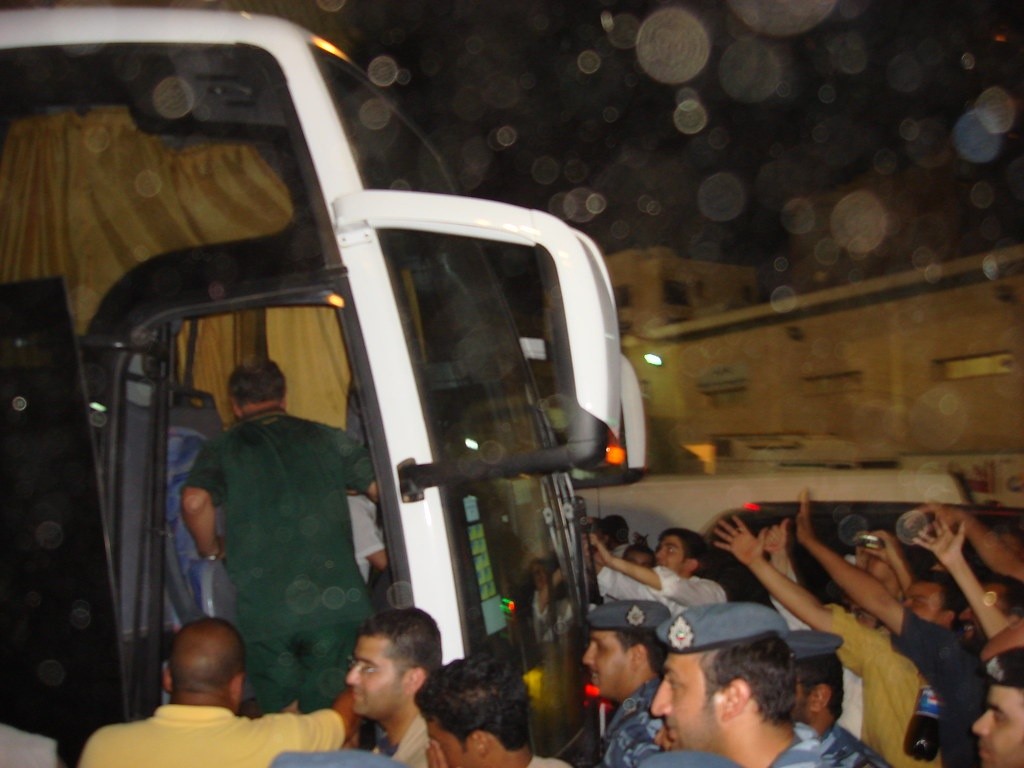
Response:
[194,544,222,561]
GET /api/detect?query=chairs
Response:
[163,388,240,632]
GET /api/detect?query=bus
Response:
[1,8,647,768]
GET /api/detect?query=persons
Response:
[0,354,1024,768]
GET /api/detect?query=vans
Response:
[516,464,972,606]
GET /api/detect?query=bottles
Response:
[901,673,942,762]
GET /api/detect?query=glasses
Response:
[346,658,413,676]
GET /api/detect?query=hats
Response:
[974,645,1024,688]
[586,599,671,628]
[784,629,844,659]
[656,603,789,653]
